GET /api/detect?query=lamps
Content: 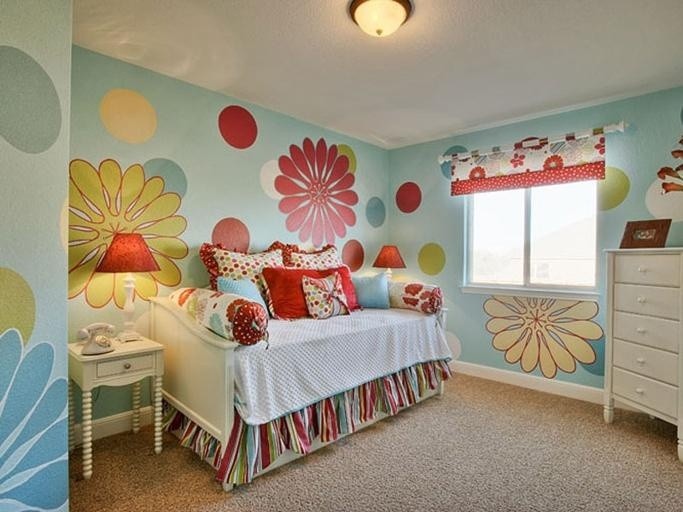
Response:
[351,0,411,38]
[95,232,161,343]
[372,246,407,273]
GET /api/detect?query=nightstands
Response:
[67,335,165,479]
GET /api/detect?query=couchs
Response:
[150,295,453,492]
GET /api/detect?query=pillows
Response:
[302,271,350,319]
[388,281,443,314]
[170,287,270,350]
[286,243,341,270]
[351,272,389,308]
[199,241,291,306]
[260,264,363,319]
[217,276,267,309]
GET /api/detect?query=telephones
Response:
[79,322,115,356]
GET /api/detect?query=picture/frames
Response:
[620,219,672,248]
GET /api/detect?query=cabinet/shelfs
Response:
[604,248,683,463]
